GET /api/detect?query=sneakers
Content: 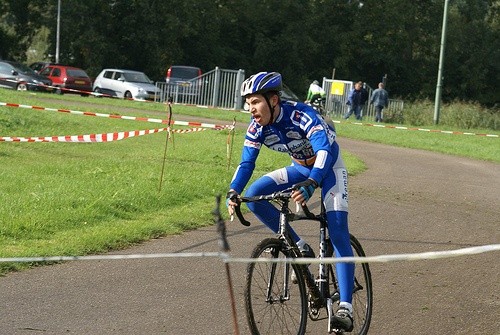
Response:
[291,242,315,284]
[331,308,353,332]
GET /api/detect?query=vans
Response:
[165,65,209,102]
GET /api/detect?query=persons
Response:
[304,80,326,107]
[345,81,389,122]
[225,72,354,333]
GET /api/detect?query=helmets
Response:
[240,72,282,97]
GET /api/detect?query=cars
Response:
[93,68,161,100]
[0,60,92,96]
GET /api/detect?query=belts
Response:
[291,155,316,166]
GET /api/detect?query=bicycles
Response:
[306,98,324,116]
[230,180,373,335]
